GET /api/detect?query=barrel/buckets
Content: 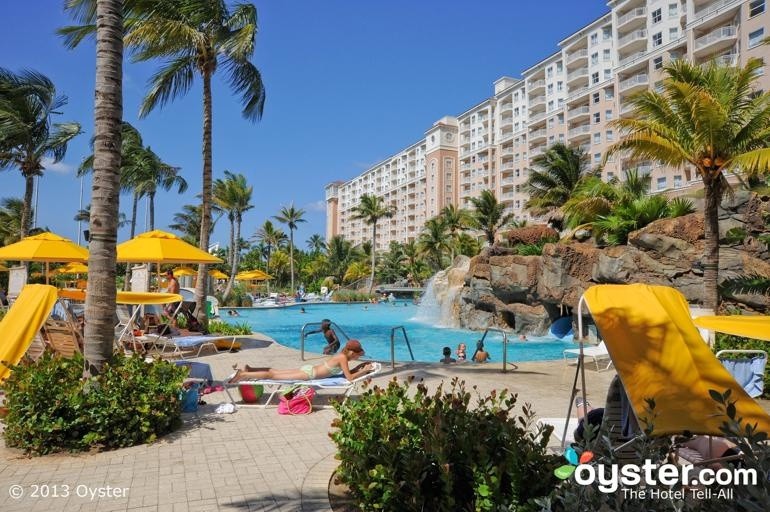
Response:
[182,383,198,411]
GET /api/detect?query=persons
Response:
[682,433,742,478]
[407,271,417,288]
[362,293,409,309]
[439,348,456,363]
[473,340,493,363]
[159,269,182,330]
[227,310,238,315]
[298,280,306,297]
[457,342,467,361]
[520,333,528,341]
[573,394,629,445]
[228,339,375,384]
[304,319,340,356]
[300,307,304,313]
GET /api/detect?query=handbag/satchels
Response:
[277,384,316,415]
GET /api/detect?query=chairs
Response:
[111,304,240,360]
[26,318,82,363]
[561,339,612,374]
[221,361,383,408]
[536,373,644,479]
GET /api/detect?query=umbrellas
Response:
[115,230,225,291]
[160,265,275,287]
[0,233,91,286]
